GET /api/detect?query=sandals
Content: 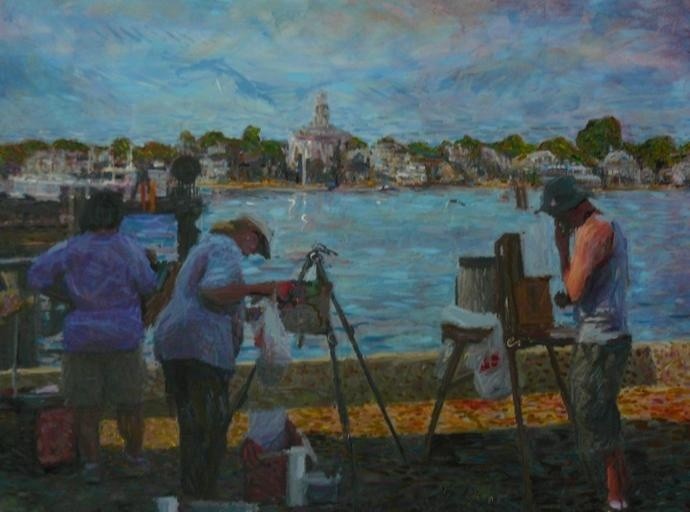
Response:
[122,459,147,475]
[83,465,101,485]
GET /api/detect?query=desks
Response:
[418,318,598,512]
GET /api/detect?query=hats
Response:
[240,211,273,260]
[534,174,592,217]
